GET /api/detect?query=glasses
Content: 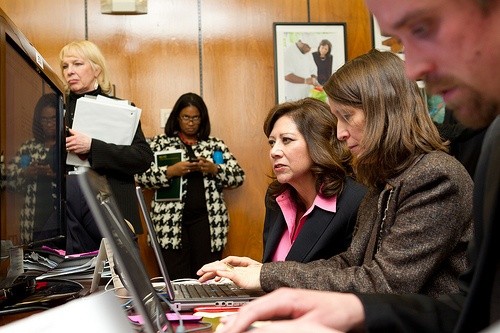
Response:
[177,115,201,124]
[0,276,36,304]
[39,116,57,126]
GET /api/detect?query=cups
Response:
[19,154,32,168]
[212,151,223,164]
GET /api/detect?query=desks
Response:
[0,280,270,333]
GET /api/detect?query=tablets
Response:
[155,149,183,201]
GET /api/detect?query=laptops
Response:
[136,187,270,312]
[77,167,174,333]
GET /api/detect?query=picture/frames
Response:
[370,12,426,88]
[272,22,348,105]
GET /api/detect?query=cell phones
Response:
[190,158,199,162]
[38,160,48,165]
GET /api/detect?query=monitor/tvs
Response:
[0,7,66,255]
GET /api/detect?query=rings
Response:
[225,262,234,270]
[188,169,190,173]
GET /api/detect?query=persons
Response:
[58,39,155,257]
[133,92,247,280]
[5,92,60,244]
[196,48,474,297]
[284,34,340,102]
[214,0,500,333]
[262,96,367,271]
